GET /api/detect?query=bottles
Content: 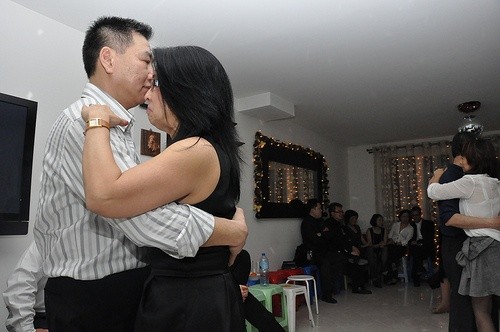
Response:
[259,253,269,286]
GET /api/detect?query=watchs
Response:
[83,117,110,135]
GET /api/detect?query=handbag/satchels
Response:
[292,243,312,266]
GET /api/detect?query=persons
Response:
[431,130,500,331]
[3,240,49,331]
[81,45,257,332]
[34,16,248,332]
[228,249,285,332]
[301,198,436,303]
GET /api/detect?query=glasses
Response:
[333,210,344,214]
[150,79,159,88]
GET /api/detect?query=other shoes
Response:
[414,275,419,287]
[322,294,338,304]
[352,286,372,293]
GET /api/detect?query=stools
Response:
[245,256,408,332]
